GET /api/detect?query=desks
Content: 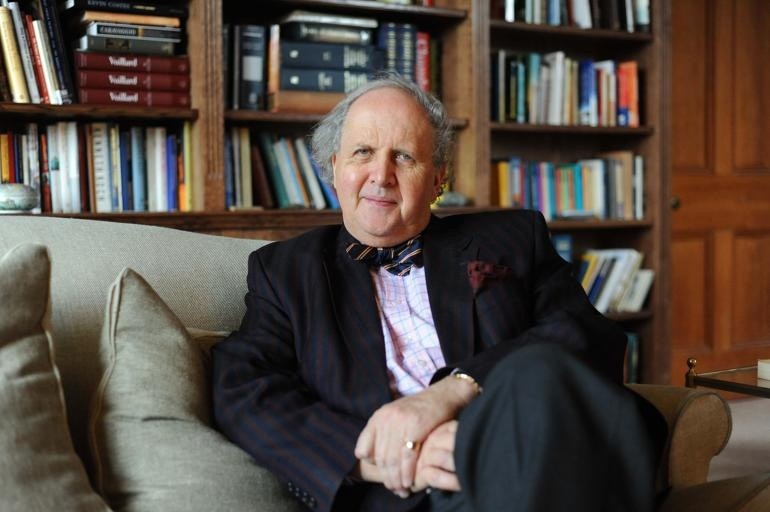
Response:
[684,354,770,399]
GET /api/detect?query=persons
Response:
[211,73,670,512]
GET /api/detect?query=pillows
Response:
[1,241,121,512]
[85,265,307,511]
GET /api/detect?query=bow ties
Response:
[345,237,422,276]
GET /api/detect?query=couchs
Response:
[1,212,770,512]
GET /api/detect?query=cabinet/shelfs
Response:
[0,0,674,387]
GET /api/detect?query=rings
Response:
[401,440,421,454]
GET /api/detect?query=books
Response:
[2,1,449,214]
[489,0,657,382]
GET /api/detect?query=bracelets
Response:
[453,371,481,399]
